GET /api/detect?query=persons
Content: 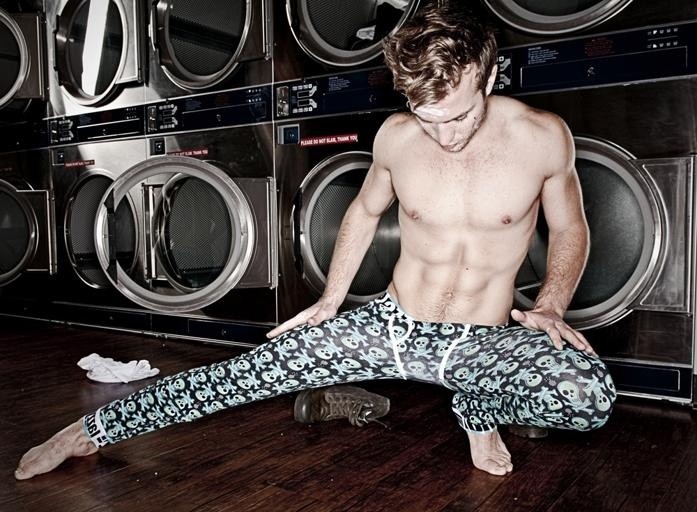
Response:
[14,2,618,481]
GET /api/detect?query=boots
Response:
[294,384,390,427]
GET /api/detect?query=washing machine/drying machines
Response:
[0,1,697,407]
